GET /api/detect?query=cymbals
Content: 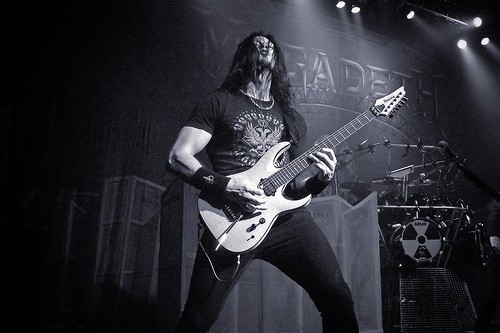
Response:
[339,182,371,190]
[407,179,437,186]
[350,187,380,200]
[372,179,408,184]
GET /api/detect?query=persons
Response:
[166,30,359,333]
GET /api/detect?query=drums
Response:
[377,190,406,219]
[434,196,456,214]
[407,191,433,214]
[391,217,442,263]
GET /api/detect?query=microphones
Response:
[439,141,461,166]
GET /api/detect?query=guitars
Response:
[197,86,409,255]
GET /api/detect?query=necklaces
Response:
[239,88,275,110]
